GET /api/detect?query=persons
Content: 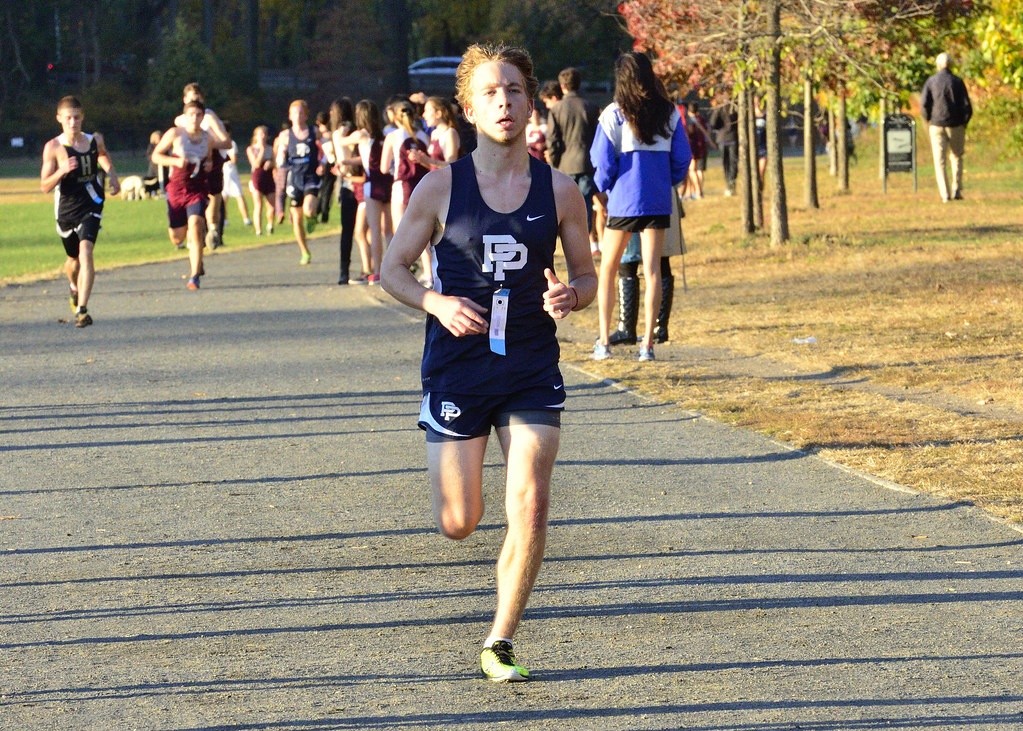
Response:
[920,53,973,203]
[380,42,599,681]
[144,51,767,362]
[40,95,120,328]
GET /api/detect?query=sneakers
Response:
[480,640,529,682]
[69,284,93,328]
[187,273,199,290]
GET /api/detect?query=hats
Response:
[936,52,950,65]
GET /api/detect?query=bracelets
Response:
[571,288,578,310]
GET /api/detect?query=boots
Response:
[637,275,674,343]
[596,277,639,345]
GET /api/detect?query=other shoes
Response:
[348,271,380,285]
[639,344,655,362]
[589,340,612,361]
[338,274,348,285]
[306,215,316,234]
[300,251,310,265]
